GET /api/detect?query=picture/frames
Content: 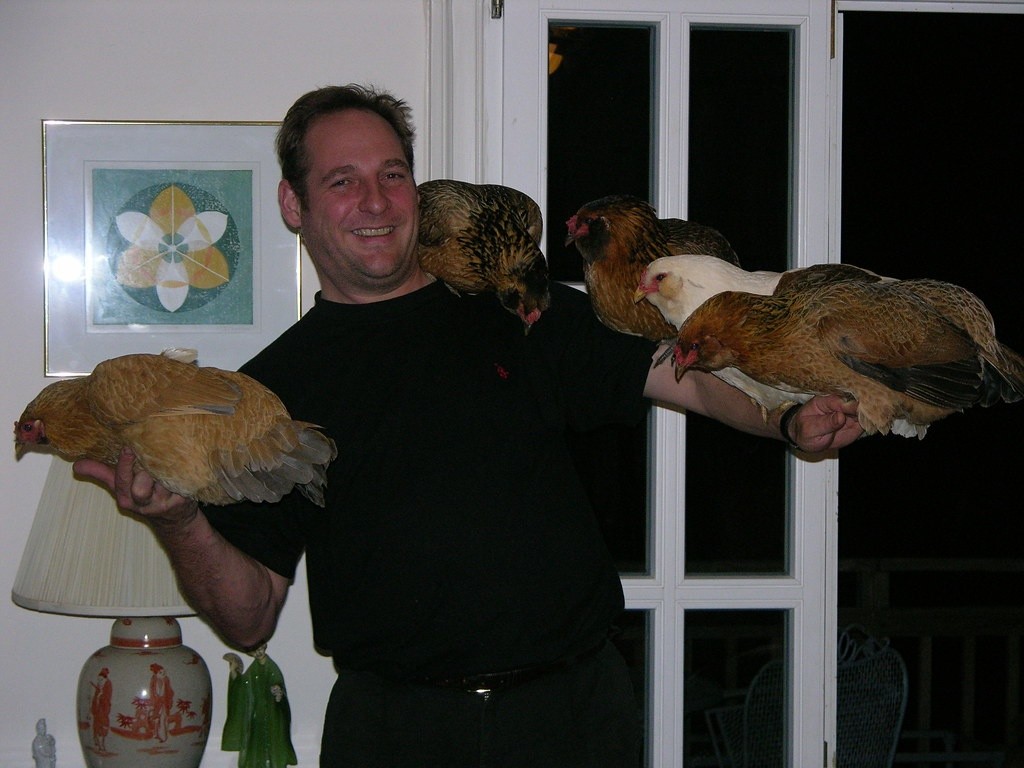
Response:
[41,118,303,379]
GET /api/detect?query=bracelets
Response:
[780,402,802,452]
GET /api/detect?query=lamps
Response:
[12,455,212,768]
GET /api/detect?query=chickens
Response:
[415,180,1024,441]
[12,351,340,509]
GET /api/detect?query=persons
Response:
[71,83,864,768]
[31,719,58,768]
[220,642,299,768]
